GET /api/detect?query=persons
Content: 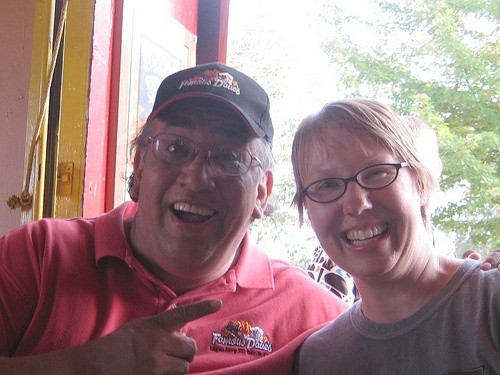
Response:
[0,62,500,375]
[291,99,500,375]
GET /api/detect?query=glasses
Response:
[301,161,409,203]
[146,133,265,176]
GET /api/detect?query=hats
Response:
[147,62,273,144]
